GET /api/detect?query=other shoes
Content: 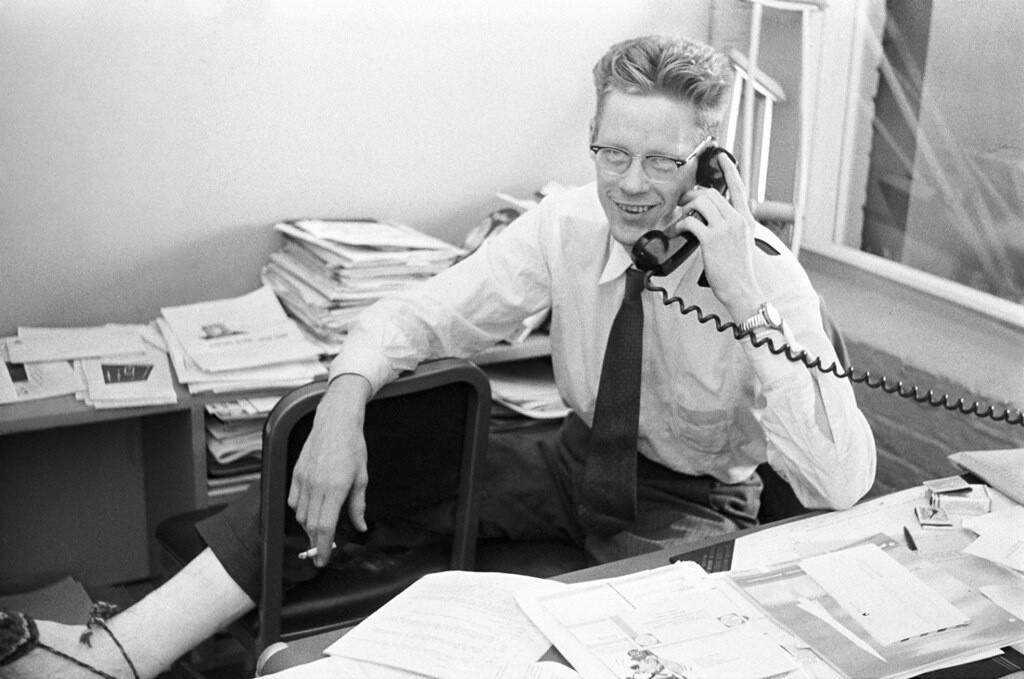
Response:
[0,610,119,679]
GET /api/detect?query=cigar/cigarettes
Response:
[298,542,337,560]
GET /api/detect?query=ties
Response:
[571,265,651,531]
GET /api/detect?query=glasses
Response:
[588,132,716,185]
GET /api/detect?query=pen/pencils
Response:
[904,527,917,550]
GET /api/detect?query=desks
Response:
[256,471,1024,679]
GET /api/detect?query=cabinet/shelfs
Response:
[0,336,551,588]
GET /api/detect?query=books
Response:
[260,212,468,347]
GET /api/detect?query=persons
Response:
[0,23,876,679]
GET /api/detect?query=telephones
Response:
[632,146,742,278]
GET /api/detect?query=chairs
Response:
[153,359,494,679]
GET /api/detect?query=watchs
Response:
[737,301,782,333]
